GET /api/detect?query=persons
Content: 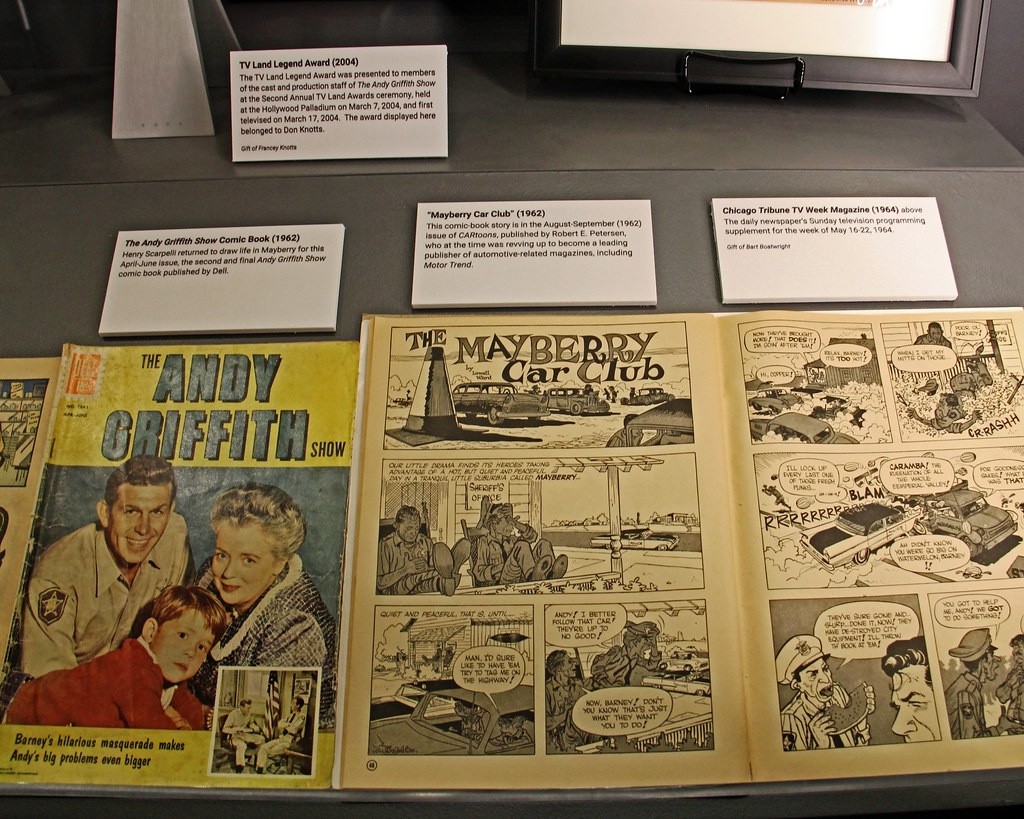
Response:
[0,453,361,786]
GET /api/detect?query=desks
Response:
[0,50,1024,819]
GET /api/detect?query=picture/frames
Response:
[524,0,992,99]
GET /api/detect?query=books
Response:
[0,356,60,677]
[3,339,359,800]
